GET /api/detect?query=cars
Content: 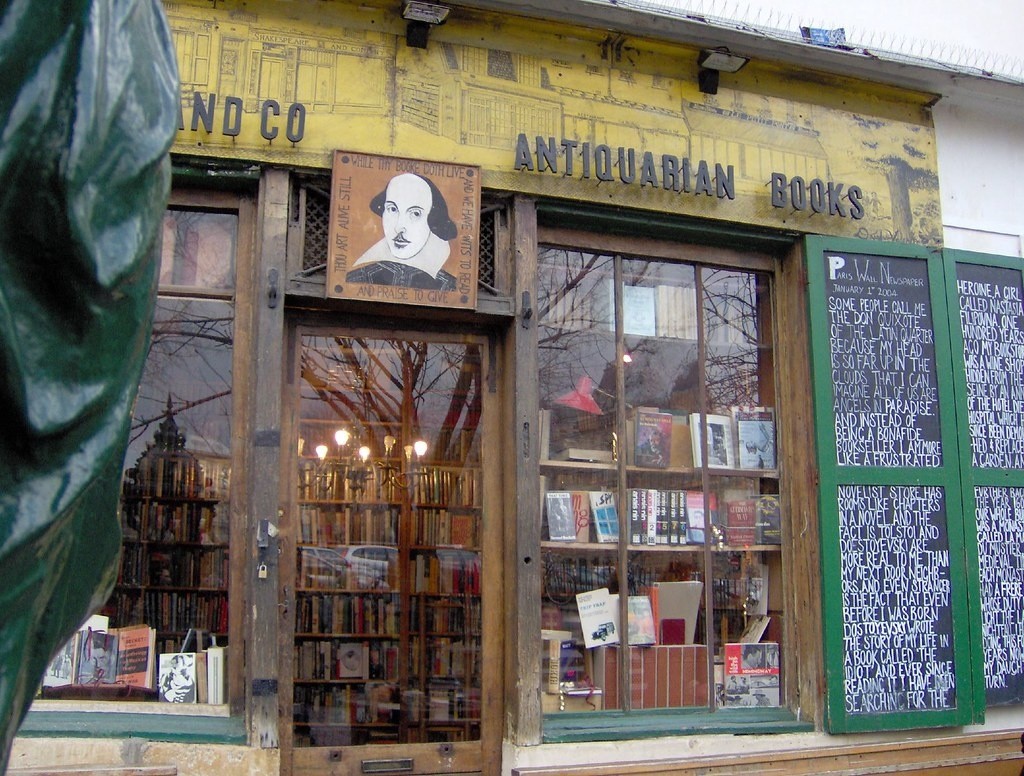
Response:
[296,543,480,589]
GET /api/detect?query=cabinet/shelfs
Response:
[107,455,779,741]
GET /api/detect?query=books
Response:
[626,474,782,548]
[628,578,709,707]
[31,611,228,706]
[540,585,621,712]
[655,283,760,344]
[295,420,485,743]
[713,561,780,708]
[536,410,613,463]
[538,266,656,337]
[627,402,777,470]
[540,473,621,544]
[109,448,227,636]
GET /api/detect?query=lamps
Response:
[296,363,433,491]
[698,46,750,95]
[551,378,632,464]
[401,0,451,49]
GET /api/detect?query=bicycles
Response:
[541,546,579,605]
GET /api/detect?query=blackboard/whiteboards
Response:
[797,235,1024,733]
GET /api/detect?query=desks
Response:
[45,681,153,703]
